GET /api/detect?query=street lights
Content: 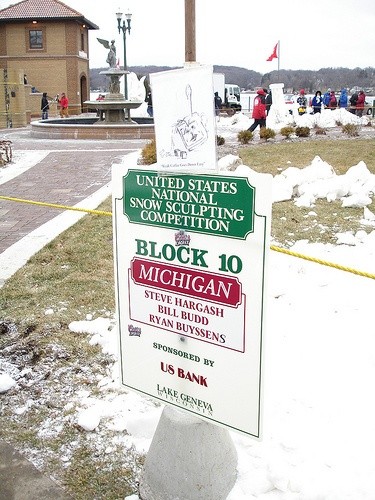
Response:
[115,6,133,117]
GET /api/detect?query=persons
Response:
[297,88,366,117]
[96,94,106,117]
[247,88,272,132]
[41,93,50,120]
[55,92,70,118]
[31,87,36,93]
[144,93,153,117]
[214,92,222,116]
[24,74,28,85]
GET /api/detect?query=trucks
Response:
[213,73,242,116]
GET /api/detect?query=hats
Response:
[327,88,330,92]
[257,90,265,95]
[300,90,304,94]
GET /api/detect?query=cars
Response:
[285,94,324,115]
[335,96,373,116]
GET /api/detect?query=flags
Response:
[266,43,278,61]
